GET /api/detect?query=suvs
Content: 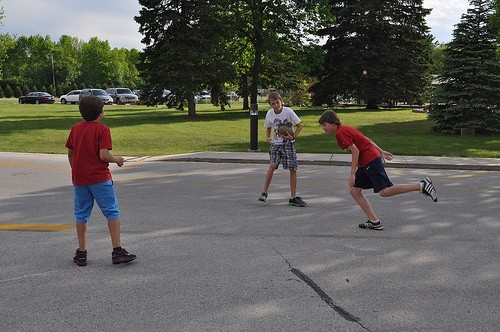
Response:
[106,88,142,106]
[79,89,114,105]
[145,89,177,106]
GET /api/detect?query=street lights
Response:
[48,49,55,92]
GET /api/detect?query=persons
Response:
[257,92,307,207]
[318,110,438,231]
[65,96,137,267]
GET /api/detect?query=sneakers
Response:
[258,192,268,202]
[420,176,437,202]
[288,196,307,207]
[112,248,136,265]
[73,248,88,266]
[359,219,383,230]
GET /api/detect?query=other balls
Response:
[277,124,294,138]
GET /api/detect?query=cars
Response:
[194,89,238,102]
[18,92,55,105]
[60,90,82,105]
